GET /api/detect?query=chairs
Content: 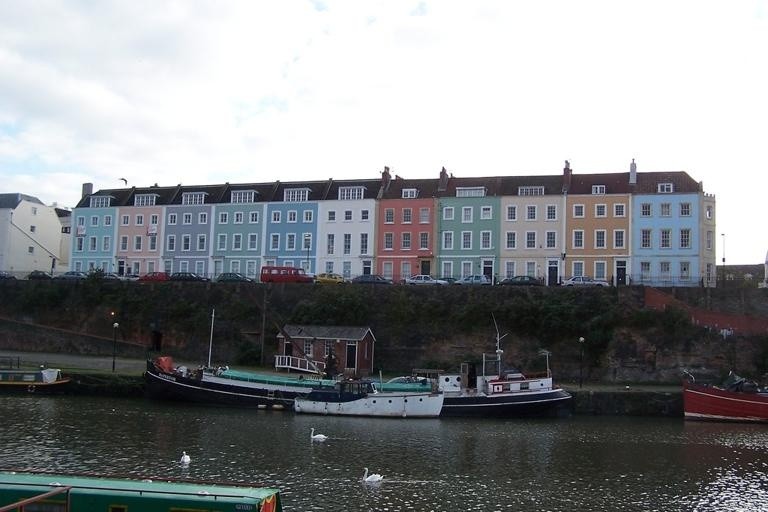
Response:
[337,367,356,380]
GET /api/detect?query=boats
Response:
[292,372,445,417]
[145,348,572,417]
[682,381,768,422]
[0,357,80,395]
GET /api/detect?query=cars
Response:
[27,270,212,281]
[217,273,255,282]
[560,275,609,289]
[313,273,492,285]
[496,276,542,286]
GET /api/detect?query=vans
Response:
[260,266,313,284]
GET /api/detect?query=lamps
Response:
[336,339,341,344]
